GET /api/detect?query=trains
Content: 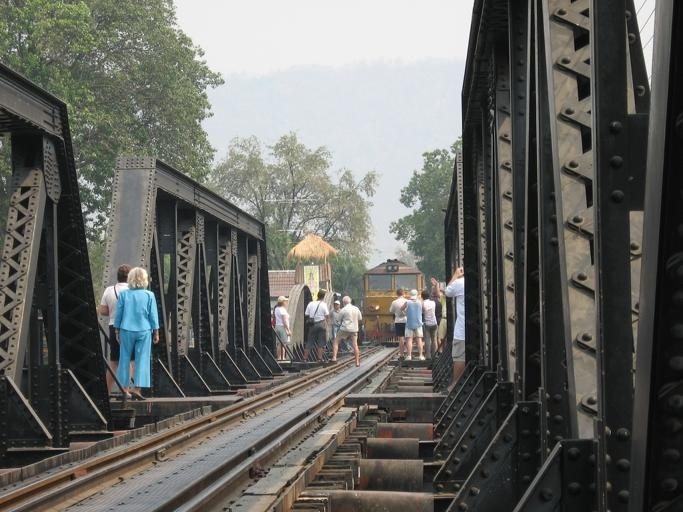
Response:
[360,258,425,346]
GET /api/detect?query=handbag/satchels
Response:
[305,318,314,326]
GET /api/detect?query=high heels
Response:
[125,392,146,400]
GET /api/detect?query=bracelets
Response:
[113,327,119,334]
[152,332,159,335]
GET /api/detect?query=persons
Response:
[388,276,447,361]
[302,290,330,362]
[327,300,345,351]
[439,257,464,394]
[98,263,135,400]
[272,295,292,361]
[112,266,161,400]
[328,295,362,367]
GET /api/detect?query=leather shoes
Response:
[329,359,336,364]
[356,359,360,367]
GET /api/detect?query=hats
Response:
[277,295,288,302]
[334,301,340,305]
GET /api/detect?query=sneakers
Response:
[400,354,434,361]
[303,358,325,363]
